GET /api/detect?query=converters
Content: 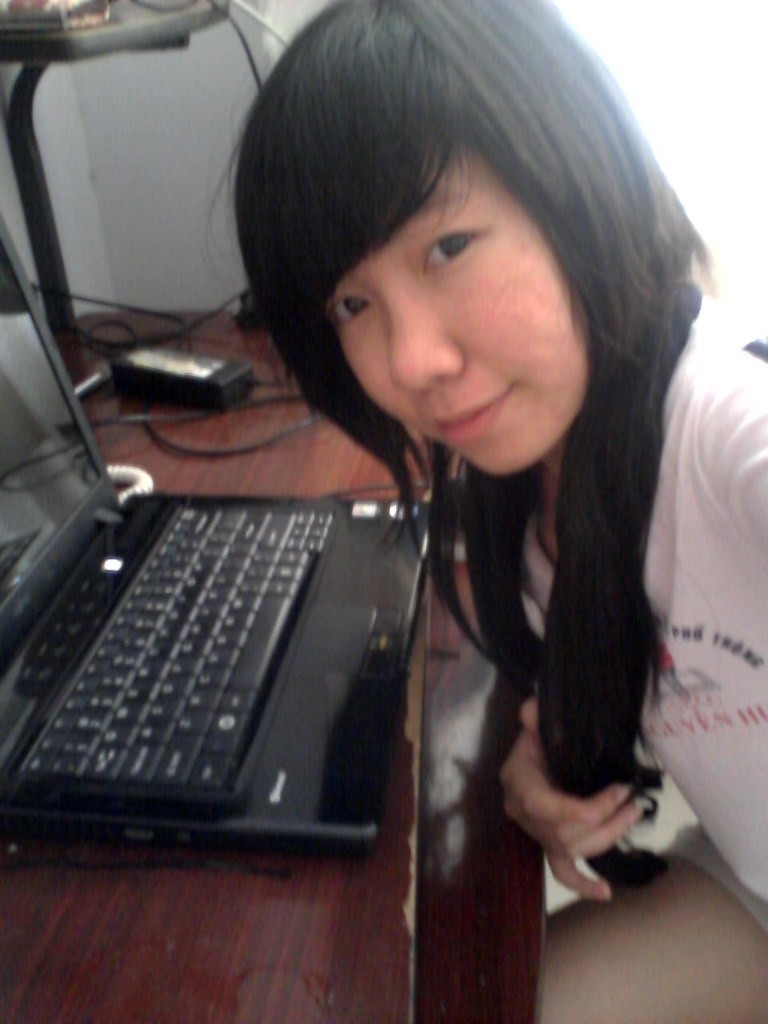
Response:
[110,344,256,412]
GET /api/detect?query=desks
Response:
[0,307,546,1024]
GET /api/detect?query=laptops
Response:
[0,215,434,850]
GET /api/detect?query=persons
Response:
[232,0,768,1024]
